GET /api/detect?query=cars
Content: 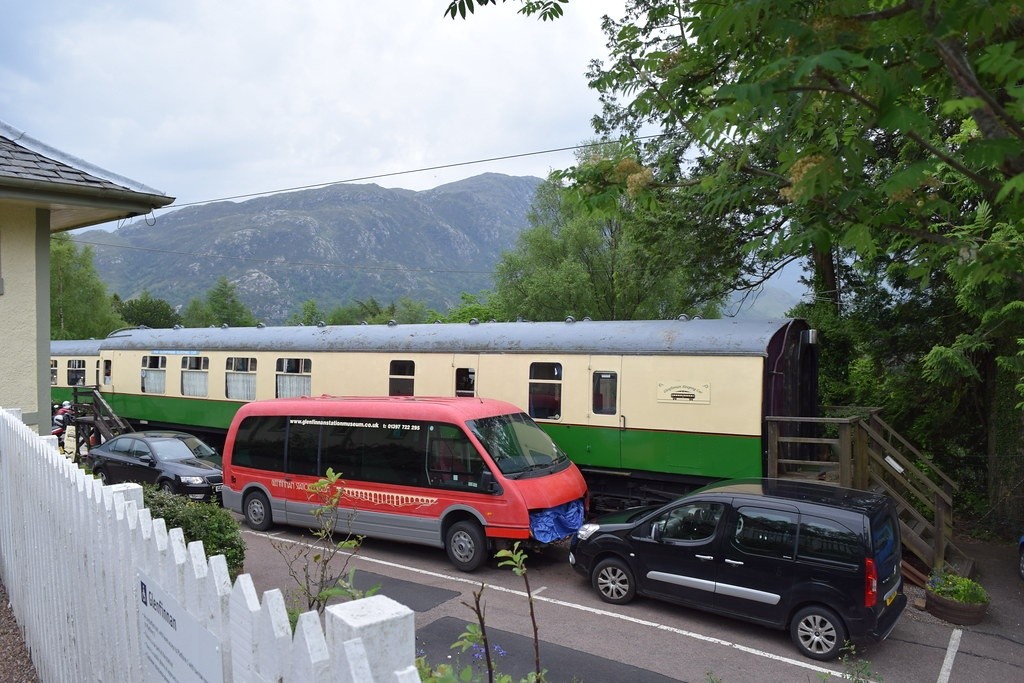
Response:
[86,431,222,502]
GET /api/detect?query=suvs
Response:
[568,477,908,662]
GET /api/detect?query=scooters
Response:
[52,401,82,439]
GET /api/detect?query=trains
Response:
[50,340,105,406]
[94,314,820,487]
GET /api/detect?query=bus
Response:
[221,394,589,572]
